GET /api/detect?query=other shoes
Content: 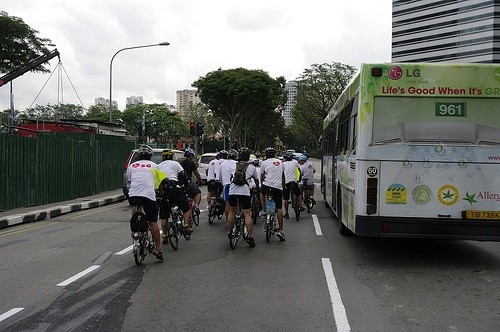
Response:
[162,234,168,244]
[288,200,291,204]
[300,206,305,212]
[228,232,233,239]
[153,249,164,260]
[277,230,286,241]
[195,207,200,214]
[206,206,209,208]
[183,224,190,232]
[246,235,255,247]
[259,212,261,216]
[283,213,289,219]
[261,210,266,219]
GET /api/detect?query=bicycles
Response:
[130,178,316,266]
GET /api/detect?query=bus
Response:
[317,62,500,242]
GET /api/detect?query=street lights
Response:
[109,42,170,123]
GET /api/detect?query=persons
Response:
[126,144,164,260]
[159,147,317,245]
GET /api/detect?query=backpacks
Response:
[233,162,249,186]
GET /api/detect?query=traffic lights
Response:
[189,122,195,136]
[196,122,204,136]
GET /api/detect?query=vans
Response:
[122,148,187,204]
[197,153,220,185]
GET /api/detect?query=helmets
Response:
[162,149,175,156]
[184,149,196,158]
[303,153,309,158]
[220,150,228,155]
[248,159,259,164]
[255,151,261,156]
[265,147,275,155]
[228,149,237,156]
[215,154,220,158]
[284,152,293,159]
[139,145,153,155]
[299,155,307,160]
[239,147,251,155]
[249,154,256,159]
